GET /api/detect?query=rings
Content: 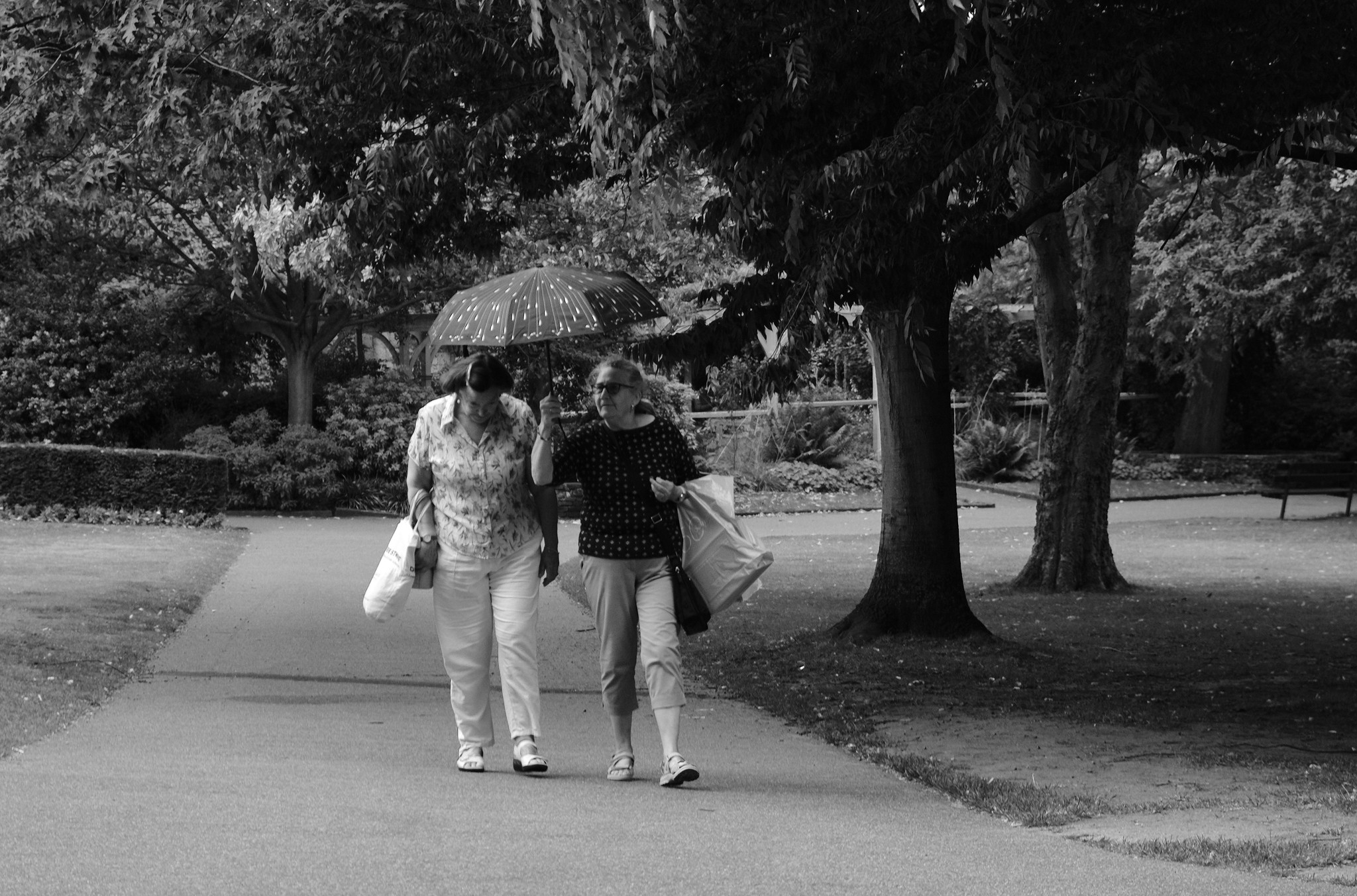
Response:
[549,408,552,415]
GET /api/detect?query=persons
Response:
[405,352,559,772]
[531,357,700,789]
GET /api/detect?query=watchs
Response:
[673,485,686,504]
[419,533,438,543]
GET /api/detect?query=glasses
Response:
[590,382,637,396]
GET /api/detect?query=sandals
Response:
[607,751,634,780]
[659,752,701,787]
[457,745,485,772]
[513,739,549,773]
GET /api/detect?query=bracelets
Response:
[537,424,554,441]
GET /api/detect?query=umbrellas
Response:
[428,263,669,424]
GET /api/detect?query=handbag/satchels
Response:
[676,474,775,614]
[671,561,711,636]
[409,490,434,589]
[363,489,431,624]
